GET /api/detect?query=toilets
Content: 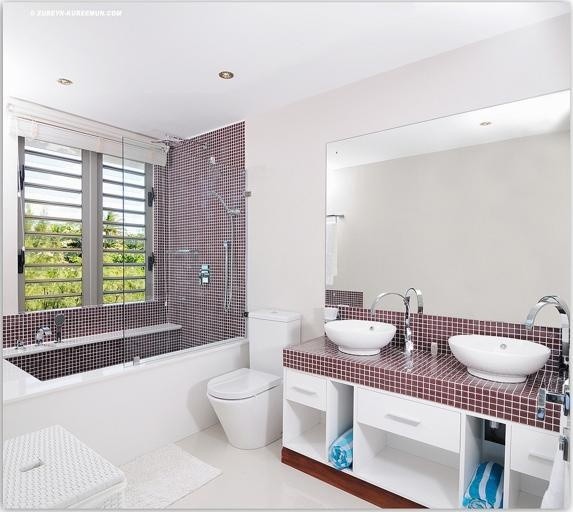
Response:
[206,311,302,450]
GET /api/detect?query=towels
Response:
[328,427,353,470]
[462,461,504,510]
[326,222,340,286]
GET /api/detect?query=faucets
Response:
[526,295,570,357]
[371,292,410,350]
[36,327,52,344]
[406,287,423,316]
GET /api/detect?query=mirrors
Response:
[324,88,571,333]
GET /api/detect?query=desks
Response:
[1,425,126,510]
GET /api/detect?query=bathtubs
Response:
[3,323,245,404]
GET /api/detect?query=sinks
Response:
[447,334,552,383]
[324,319,396,355]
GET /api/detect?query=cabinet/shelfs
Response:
[281,367,560,509]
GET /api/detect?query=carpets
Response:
[114,441,223,508]
